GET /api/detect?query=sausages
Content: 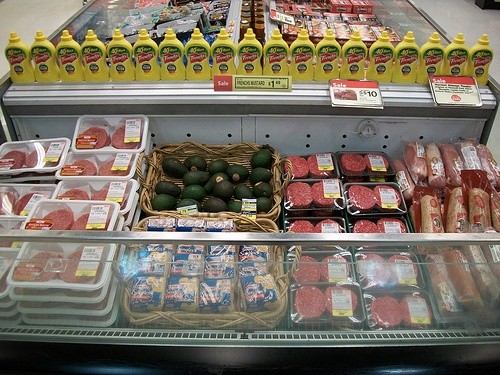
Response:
[285,141,500,328]
[0,126,138,321]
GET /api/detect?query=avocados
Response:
[152,150,273,213]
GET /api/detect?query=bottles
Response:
[31,31,60,84]
[240,0,264,44]
[289,29,315,84]
[367,32,394,83]
[262,29,289,77]
[5,32,35,83]
[132,28,160,82]
[184,28,210,81]
[81,30,109,82]
[107,30,135,82]
[314,29,342,82]
[56,30,85,82]
[238,29,263,77]
[416,33,446,84]
[392,31,419,84]
[210,27,238,78]
[466,34,493,86]
[340,31,367,81]
[157,29,186,82]
[441,33,468,76]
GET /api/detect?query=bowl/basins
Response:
[0,114,151,328]
[280,152,435,329]
